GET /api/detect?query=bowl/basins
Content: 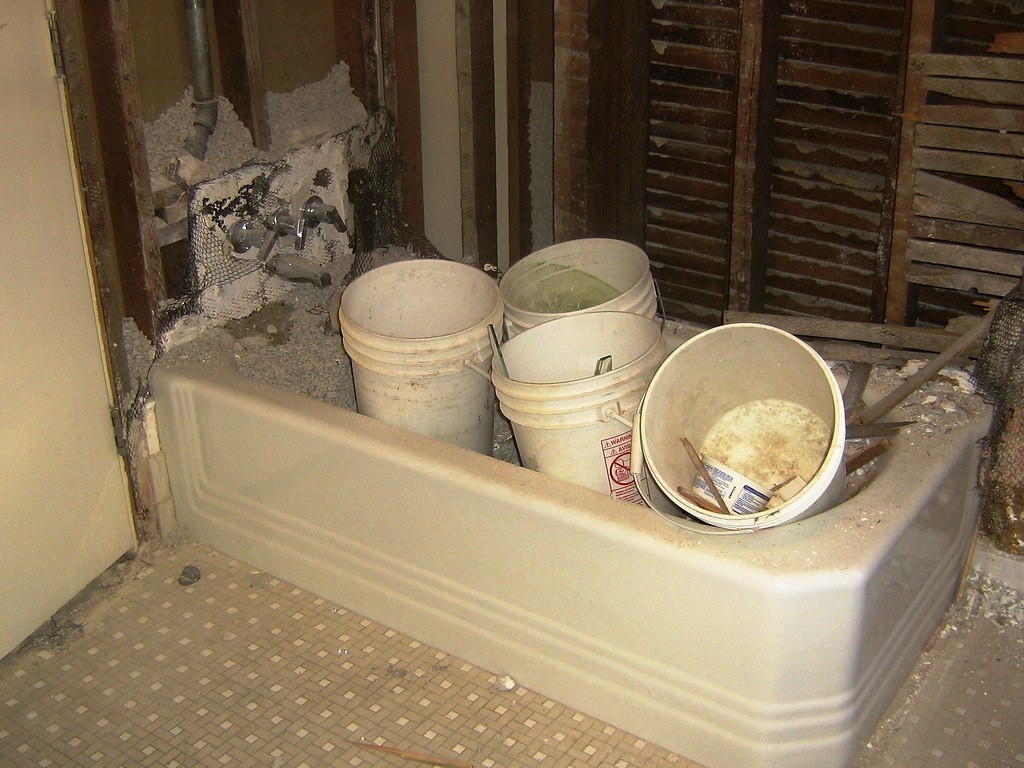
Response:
[691,453,778,518]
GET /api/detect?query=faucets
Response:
[263,251,333,290]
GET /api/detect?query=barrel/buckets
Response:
[338,234,683,522]
[629,322,847,536]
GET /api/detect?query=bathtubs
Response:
[148,240,993,768]
